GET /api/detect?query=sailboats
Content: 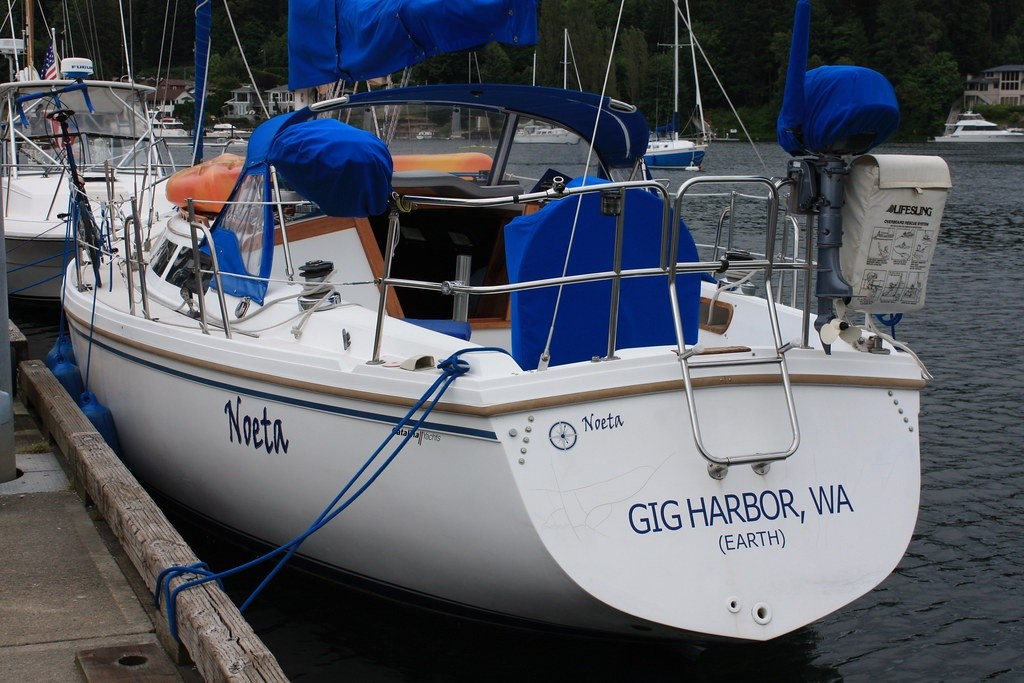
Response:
[640,0,709,173]
[0,0,207,306]
[35,0,952,649]
[512,27,585,145]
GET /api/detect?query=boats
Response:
[933,110,1024,144]
[112,108,253,148]
